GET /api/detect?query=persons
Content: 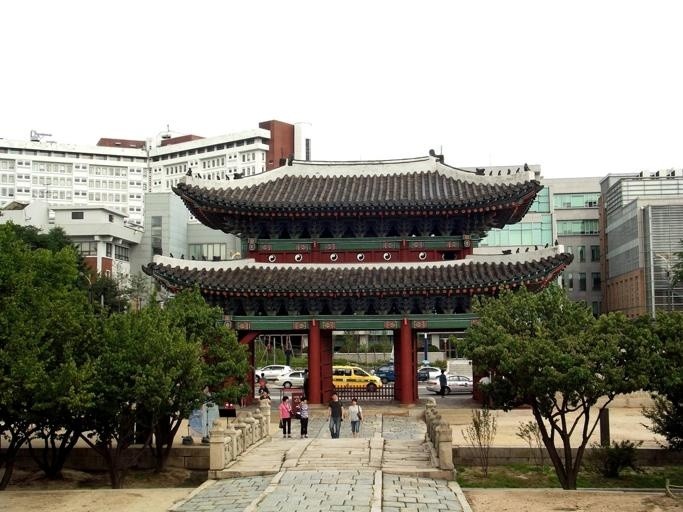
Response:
[302,369,308,400]
[439,369,446,398]
[327,393,345,438]
[258,373,267,393]
[259,392,270,400]
[284,346,291,364]
[347,398,363,437]
[295,397,308,438]
[476,371,491,408]
[278,396,293,438]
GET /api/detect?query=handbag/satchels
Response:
[279,419,284,428]
[290,410,295,418]
[357,412,361,420]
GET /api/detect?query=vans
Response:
[332,365,382,392]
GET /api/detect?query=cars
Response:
[254,364,291,383]
[425,374,473,395]
[278,369,308,388]
[417,366,441,380]
[375,364,427,384]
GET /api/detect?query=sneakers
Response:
[284,435,286,438]
[301,437,304,438]
[288,436,291,437]
[305,436,308,438]
